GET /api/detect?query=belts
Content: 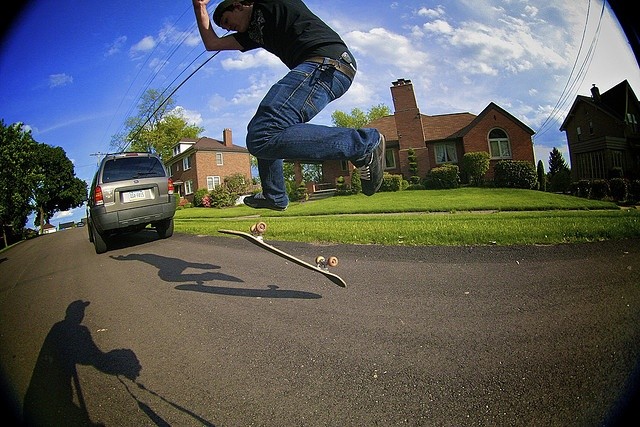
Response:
[305,57,354,82]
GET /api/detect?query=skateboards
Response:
[218,222,348,288]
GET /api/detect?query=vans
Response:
[85,151,178,254]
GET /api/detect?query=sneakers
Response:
[244,190,286,211]
[356,134,385,196]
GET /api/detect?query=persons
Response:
[192,0,386,212]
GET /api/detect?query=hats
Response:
[213,0,244,27]
